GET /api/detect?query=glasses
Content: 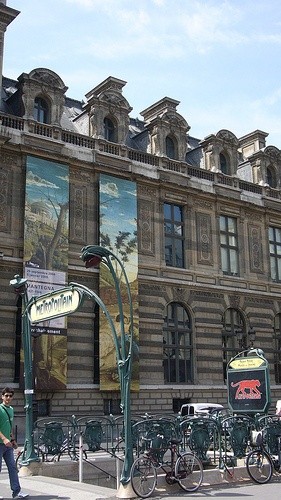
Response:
[4,395,12,397]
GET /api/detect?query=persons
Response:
[0,386,29,500]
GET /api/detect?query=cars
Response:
[178,402,232,435]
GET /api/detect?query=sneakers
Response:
[13,492,29,500]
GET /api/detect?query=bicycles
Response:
[16,431,87,473]
[245,428,281,484]
[130,438,204,499]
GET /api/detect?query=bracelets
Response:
[11,439,14,441]
